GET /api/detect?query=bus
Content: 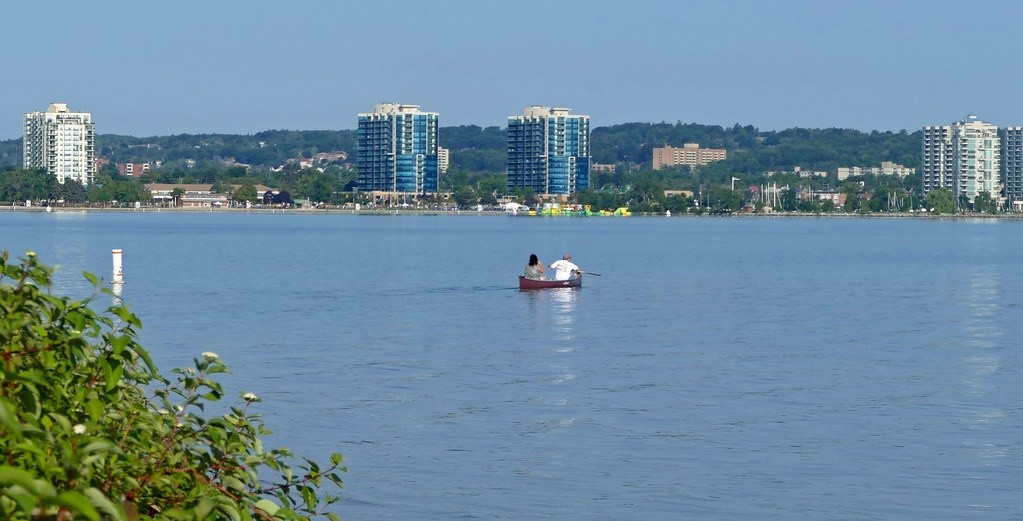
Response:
[564,205,582,211]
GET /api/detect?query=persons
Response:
[549,254,580,281]
[524,254,546,280]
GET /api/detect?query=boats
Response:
[519,272,582,289]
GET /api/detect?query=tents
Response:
[214,201,222,207]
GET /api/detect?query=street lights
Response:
[731,177,740,190]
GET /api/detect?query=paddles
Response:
[581,271,602,277]
